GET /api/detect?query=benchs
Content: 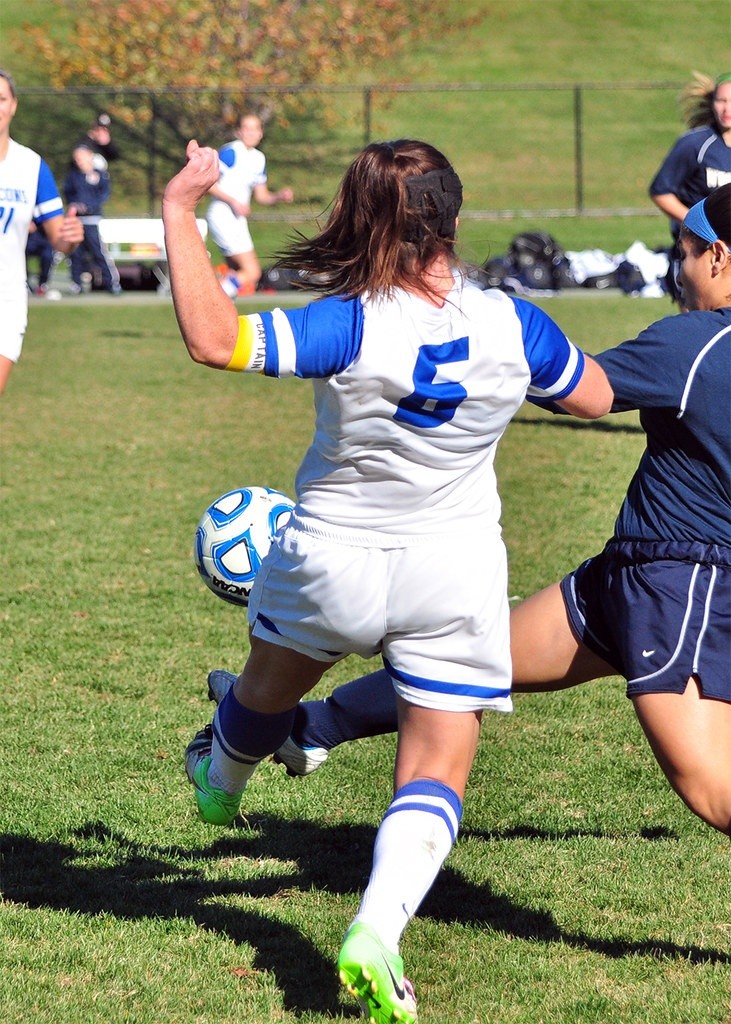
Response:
[92,214,210,297]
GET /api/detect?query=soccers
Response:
[191,484,297,609]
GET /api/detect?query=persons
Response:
[210,184,731,839]
[25,221,58,298]
[0,69,84,393]
[207,113,294,300]
[649,71,731,313]
[162,139,615,1024]
[63,114,123,296]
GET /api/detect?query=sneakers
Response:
[333,922,419,1024]
[207,669,330,777]
[183,730,245,826]
